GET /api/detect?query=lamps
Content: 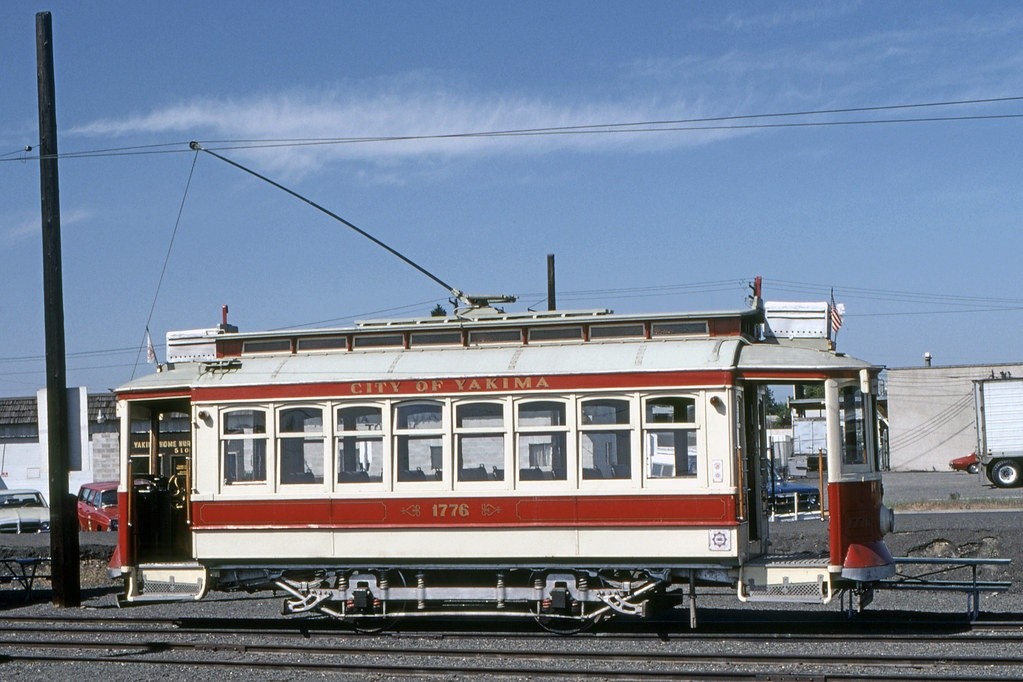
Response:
[97,409,107,424]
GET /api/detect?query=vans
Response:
[77,481,122,532]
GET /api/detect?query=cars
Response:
[0,489,52,535]
[762,458,820,512]
[950,452,979,474]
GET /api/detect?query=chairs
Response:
[583,465,603,480]
[492,466,504,481]
[611,464,631,479]
[282,469,315,483]
[551,467,567,480]
[520,464,544,480]
[338,468,370,482]
[398,467,426,481]
[435,467,442,481]
[459,463,488,481]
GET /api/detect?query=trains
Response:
[106,278,897,636]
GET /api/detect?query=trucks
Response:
[972,377,1023,488]
